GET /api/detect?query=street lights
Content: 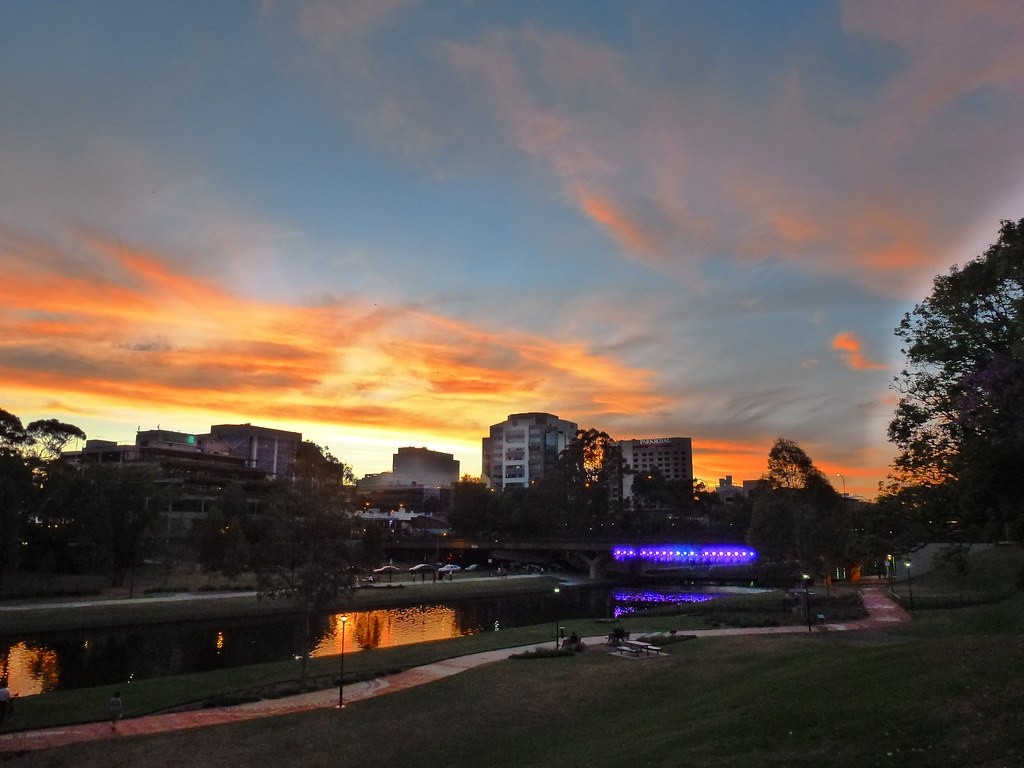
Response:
[904,558,914,612]
[836,473,845,498]
[436,531,447,563]
[554,585,560,650]
[801,571,811,634]
[339,614,348,708]
[887,554,894,593]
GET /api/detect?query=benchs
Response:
[562,638,581,648]
[617,647,639,657]
[609,632,630,641]
[639,646,661,656]
[361,578,376,583]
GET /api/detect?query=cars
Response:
[437,565,462,573]
[341,565,367,575]
[408,563,438,573]
[374,566,400,574]
[465,563,481,572]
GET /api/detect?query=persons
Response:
[449,570,452,581]
[612,625,624,642]
[567,631,578,645]
[368,574,374,583]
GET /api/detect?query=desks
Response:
[626,641,652,655]
